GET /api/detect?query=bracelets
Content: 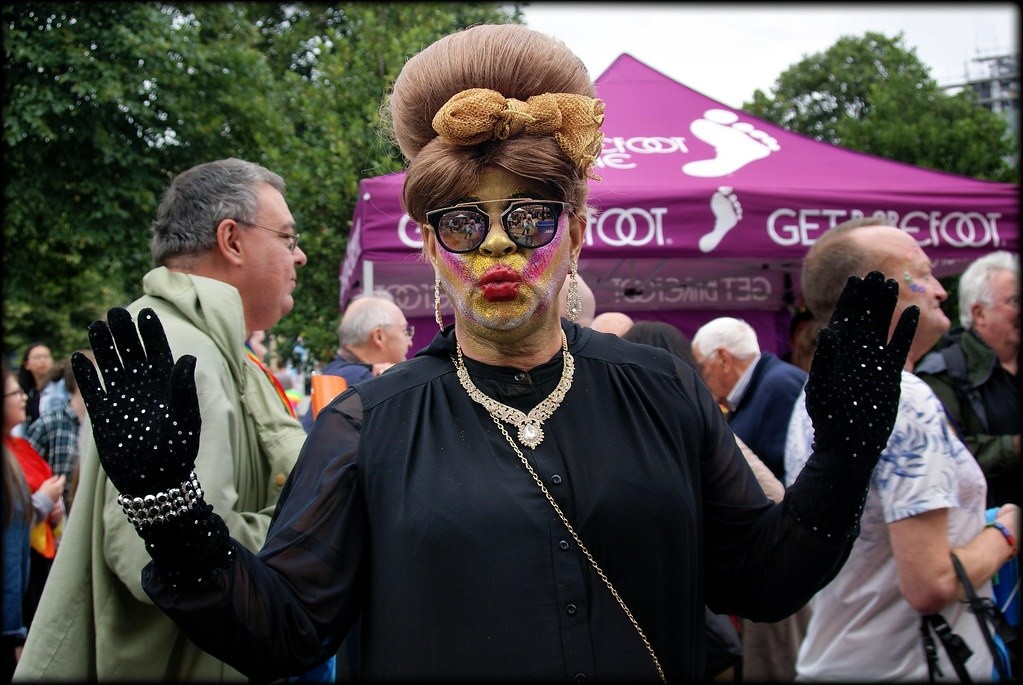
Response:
[118,471,205,528]
[984,521,1018,560]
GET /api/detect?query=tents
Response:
[339,53,1023,366]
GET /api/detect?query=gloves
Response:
[787,270,920,538]
[71,308,234,591]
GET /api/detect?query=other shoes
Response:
[531,244,534,247]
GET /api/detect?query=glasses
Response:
[426,197,575,253]
[3,388,23,401]
[369,324,415,339]
[216,216,299,254]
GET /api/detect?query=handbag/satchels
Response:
[464,234,467,240]
[521,230,525,236]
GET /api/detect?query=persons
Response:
[439,211,485,251]
[507,205,554,246]
[557,216,1023,685]
[0,159,413,685]
[70,26,922,685]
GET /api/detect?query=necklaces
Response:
[457,328,575,451]
